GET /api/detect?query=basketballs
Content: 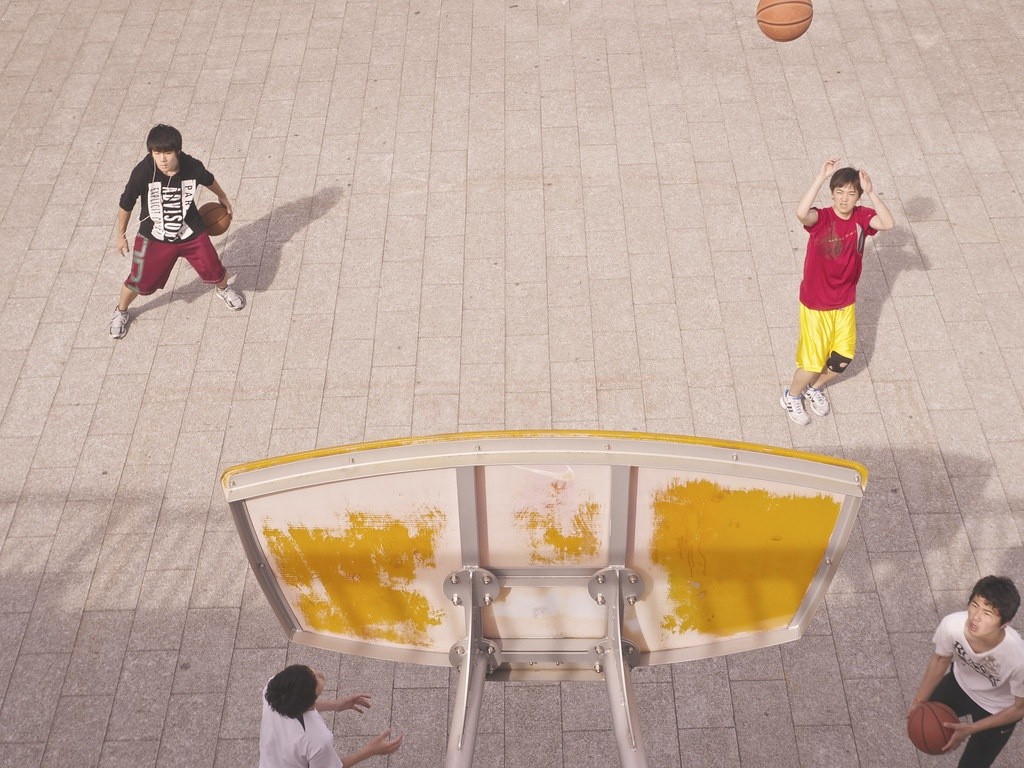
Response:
[907,702,960,756]
[199,202,231,235]
[756,0,813,43]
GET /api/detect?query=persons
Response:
[779,157,895,426]
[109,124,245,339]
[907,575,1024,768]
[259,664,402,768]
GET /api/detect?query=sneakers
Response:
[215,285,243,310]
[801,382,830,417]
[780,389,810,425]
[109,305,129,337]
